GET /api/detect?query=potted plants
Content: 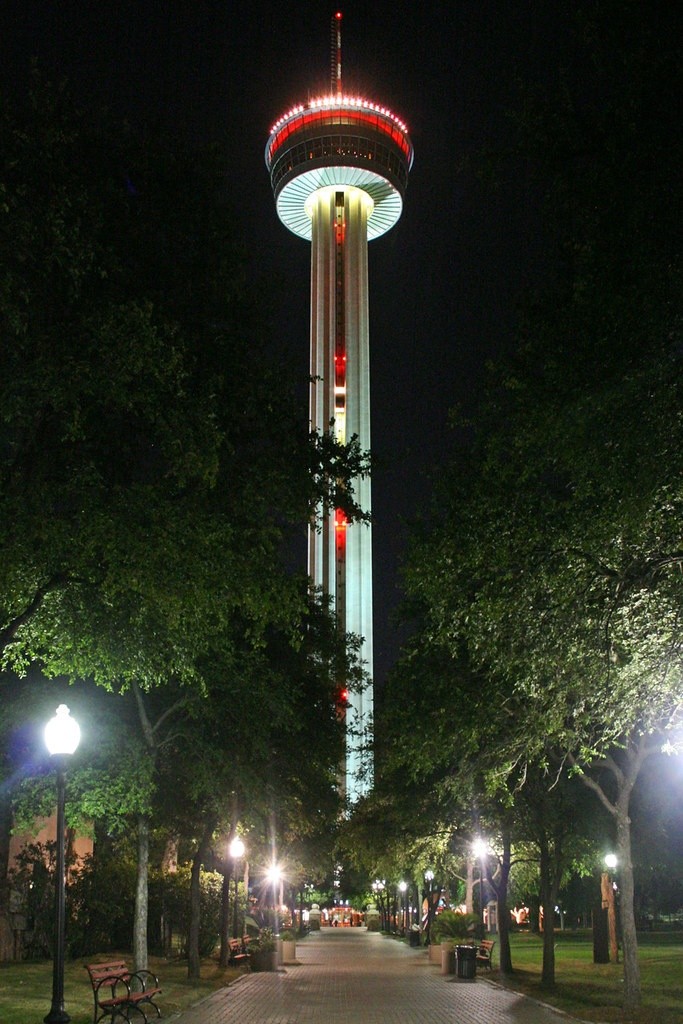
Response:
[428,933,443,965]
[278,929,296,961]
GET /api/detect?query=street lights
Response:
[42,704,82,1023]
[399,880,408,931]
[605,853,625,948]
[229,835,246,939]
[473,838,487,942]
[424,869,436,944]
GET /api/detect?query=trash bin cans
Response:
[409,929,420,947]
[452,943,478,979]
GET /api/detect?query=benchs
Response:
[82,959,162,1023]
[227,933,252,965]
[475,939,496,973]
